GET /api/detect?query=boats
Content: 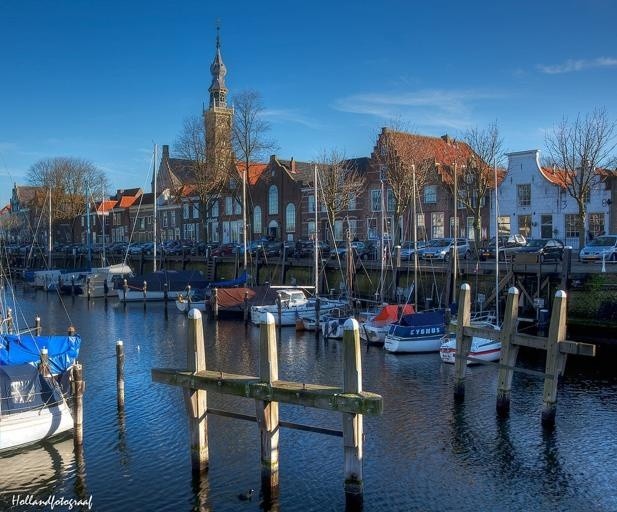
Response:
[0,362,75,454]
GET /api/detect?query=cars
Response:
[10,229,617,263]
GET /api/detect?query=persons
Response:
[289,274,297,286]
[296,239,302,260]
[397,304,409,326]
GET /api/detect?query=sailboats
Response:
[23,143,520,366]
